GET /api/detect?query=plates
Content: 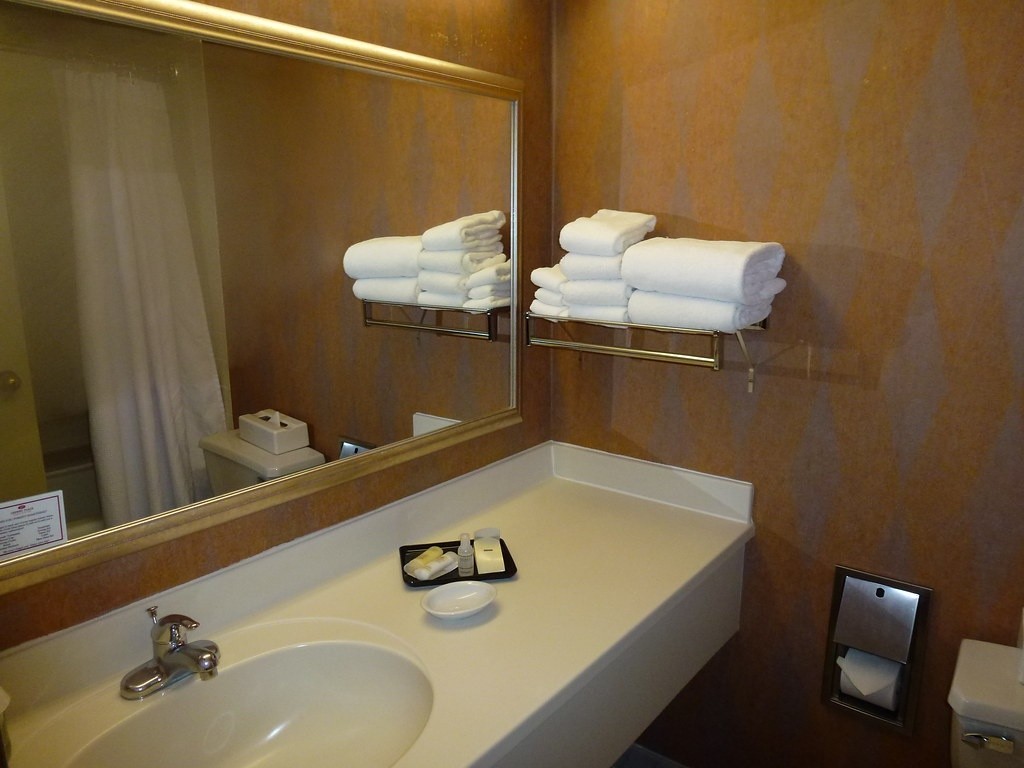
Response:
[421,581,496,619]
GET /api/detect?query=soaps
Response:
[473,537,507,575]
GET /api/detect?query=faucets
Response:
[118,612,222,701]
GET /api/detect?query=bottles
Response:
[458,534,474,577]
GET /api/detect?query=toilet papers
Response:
[836,647,903,712]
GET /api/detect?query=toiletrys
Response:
[403,532,475,582]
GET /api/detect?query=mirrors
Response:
[0,2,526,598]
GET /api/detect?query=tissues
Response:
[239,408,311,455]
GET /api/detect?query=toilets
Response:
[946,636,1024,768]
[197,428,326,498]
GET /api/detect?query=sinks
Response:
[60,637,437,768]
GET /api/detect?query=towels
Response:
[526,208,789,336]
[342,209,512,311]
